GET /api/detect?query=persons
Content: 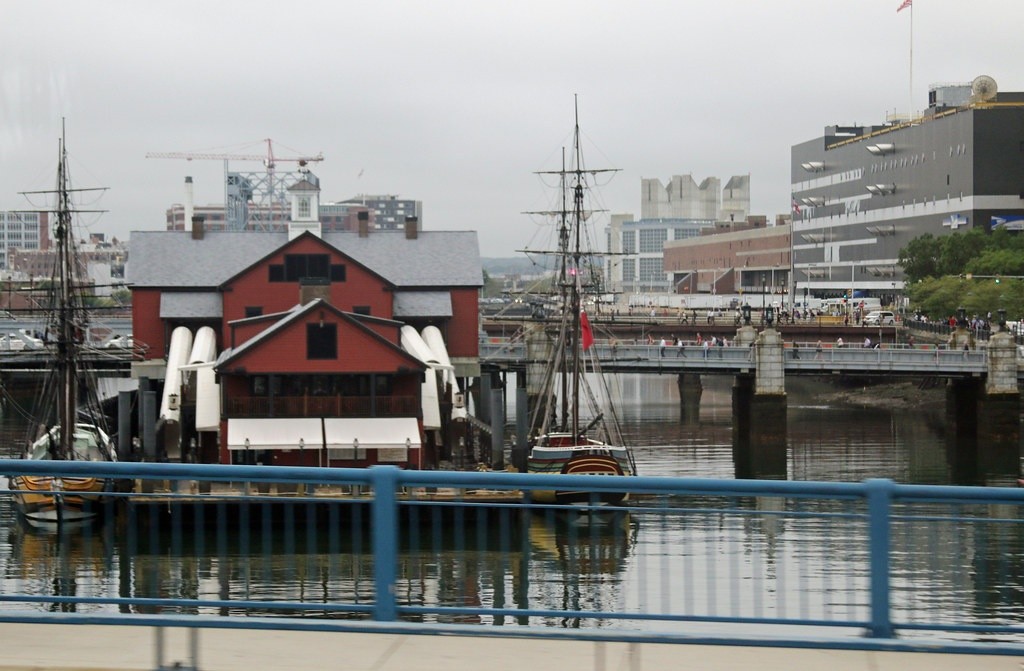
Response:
[593,300,1024,359]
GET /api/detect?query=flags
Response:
[580,306,594,352]
[792,196,801,214]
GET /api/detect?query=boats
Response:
[0,323,133,352]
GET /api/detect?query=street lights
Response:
[7,275,11,316]
[781,277,785,310]
[29,274,34,314]
[761,273,766,314]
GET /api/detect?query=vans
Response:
[863,310,895,326]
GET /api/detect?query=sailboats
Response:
[495,93,638,507]
[0,116,118,523]
